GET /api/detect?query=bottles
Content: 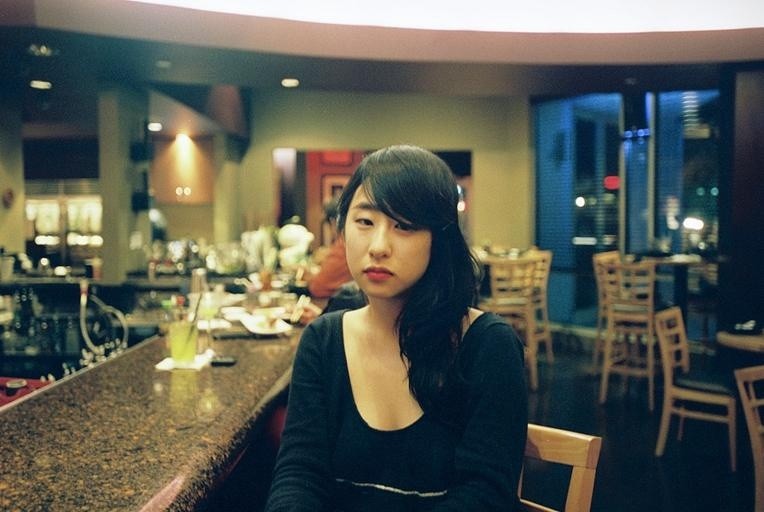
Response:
[191,268,212,355]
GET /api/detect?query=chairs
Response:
[472,249,554,391]
[653,306,737,469]
[516,420,602,510]
[593,250,703,403]
[735,365,763,511]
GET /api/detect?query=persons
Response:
[267,146,529,512]
[294,281,370,327]
[291,199,354,298]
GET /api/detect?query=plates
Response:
[240,314,292,335]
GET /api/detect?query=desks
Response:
[718,328,762,356]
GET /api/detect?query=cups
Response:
[169,308,198,362]
